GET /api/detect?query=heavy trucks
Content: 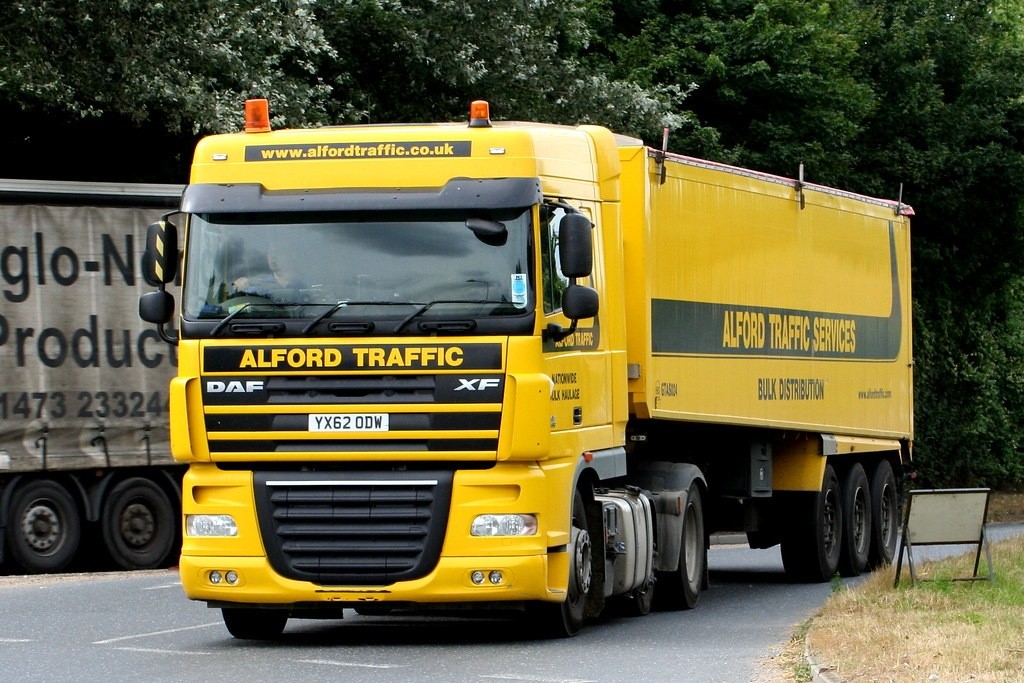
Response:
[169,98,915,641]
[0,178,188,571]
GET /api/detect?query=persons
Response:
[232,236,313,306]
